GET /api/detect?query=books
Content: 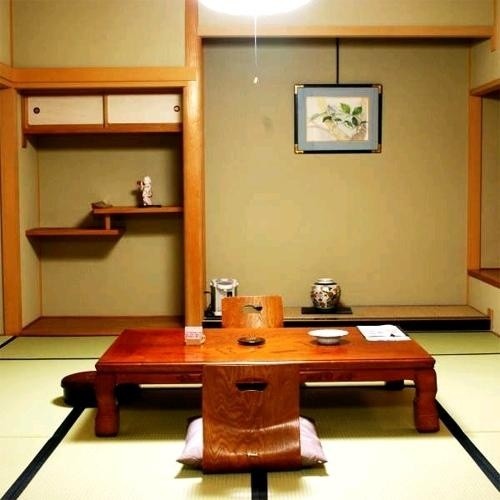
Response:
[355,325,411,342]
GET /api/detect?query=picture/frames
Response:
[294,82,383,154]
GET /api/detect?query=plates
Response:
[306,329,349,346]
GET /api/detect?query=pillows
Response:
[176,416,327,468]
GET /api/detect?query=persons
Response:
[140,176,153,205]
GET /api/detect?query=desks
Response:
[95,327,440,439]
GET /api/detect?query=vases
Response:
[311,279,341,310]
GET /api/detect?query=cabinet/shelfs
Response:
[1,64,205,337]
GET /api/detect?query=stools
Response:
[62,371,140,405]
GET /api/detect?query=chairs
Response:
[222,296,284,327]
[201,364,302,473]
